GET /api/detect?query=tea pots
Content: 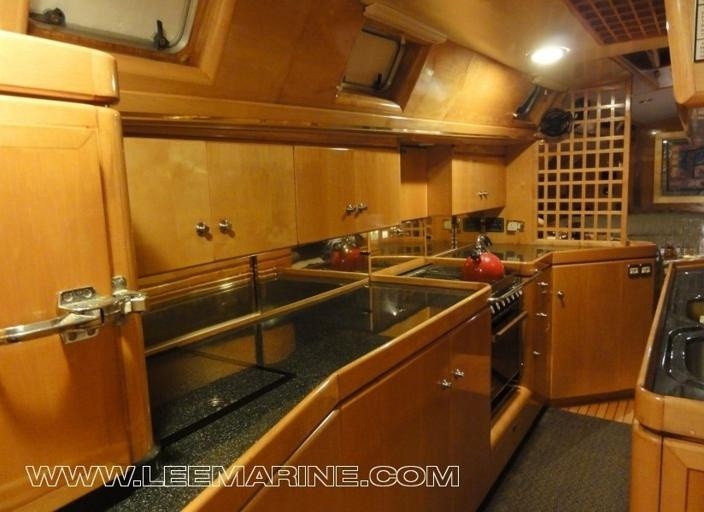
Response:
[330,236,360,267]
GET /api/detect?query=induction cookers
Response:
[402,265,521,330]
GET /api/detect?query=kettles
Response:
[462,234,505,282]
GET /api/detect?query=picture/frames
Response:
[654,132,704,205]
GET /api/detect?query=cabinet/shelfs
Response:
[630,417,704,512]
[0,29,157,512]
[120,136,507,280]
[240,258,655,512]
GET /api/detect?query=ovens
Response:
[490,300,529,422]
